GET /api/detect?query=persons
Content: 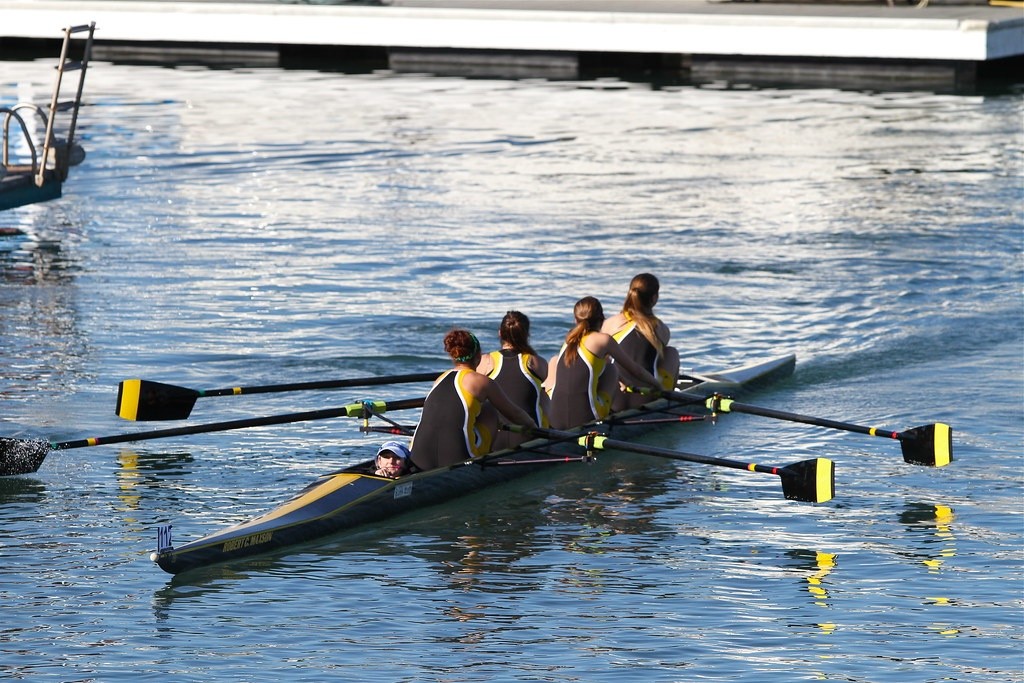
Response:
[374,441,409,479]
[408,272,680,472]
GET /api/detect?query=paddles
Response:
[0,397,427,478]
[496,421,837,505]
[624,385,955,468]
[115,370,449,423]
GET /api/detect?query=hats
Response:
[376,441,408,459]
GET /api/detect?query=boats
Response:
[150,354,797,575]
[0,21,98,212]
